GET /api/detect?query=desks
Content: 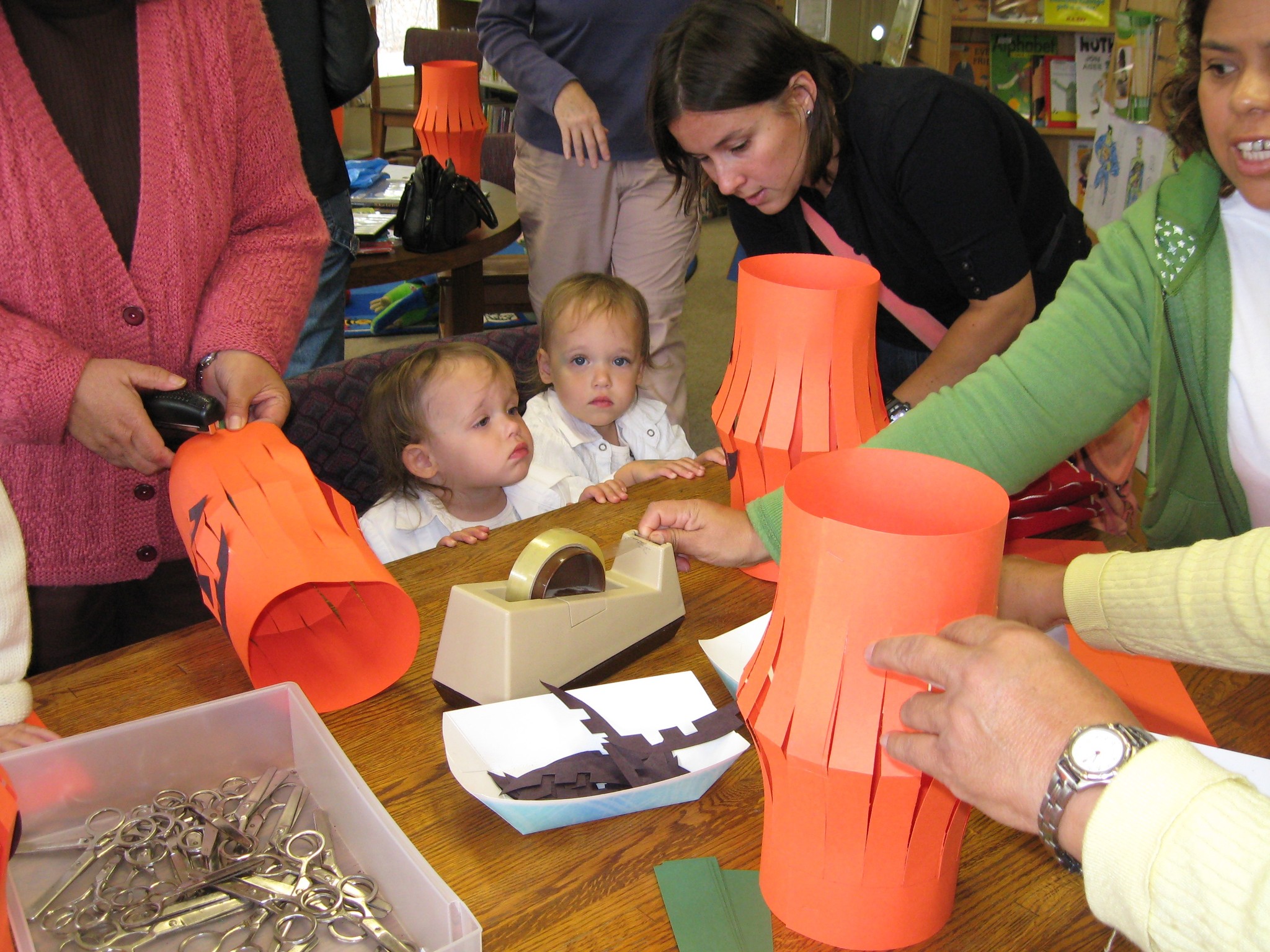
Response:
[28,460,1270,952]
[349,164,522,338]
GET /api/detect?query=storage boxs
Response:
[0,680,483,952]
[1037,0,1111,27]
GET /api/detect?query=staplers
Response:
[140,389,226,452]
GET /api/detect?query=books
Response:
[949,0,1165,137]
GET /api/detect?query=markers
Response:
[351,202,374,207]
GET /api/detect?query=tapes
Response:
[506,528,650,602]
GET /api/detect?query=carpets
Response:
[343,239,538,338]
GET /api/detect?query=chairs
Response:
[370,28,483,159]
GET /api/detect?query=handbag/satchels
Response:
[393,154,498,256]
[1065,397,1150,535]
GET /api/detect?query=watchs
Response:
[197,352,217,391]
[1040,712,1170,872]
[883,392,910,424]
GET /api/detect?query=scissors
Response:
[14,767,416,952]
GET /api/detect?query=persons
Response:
[645,1,1095,437]
[495,266,731,503]
[861,493,1270,952]
[2,480,72,759]
[0,1,334,677]
[471,0,764,448]
[336,339,627,566]
[639,2,1270,554]
[262,0,380,388]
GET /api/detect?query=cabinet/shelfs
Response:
[952,20,1113,137]
